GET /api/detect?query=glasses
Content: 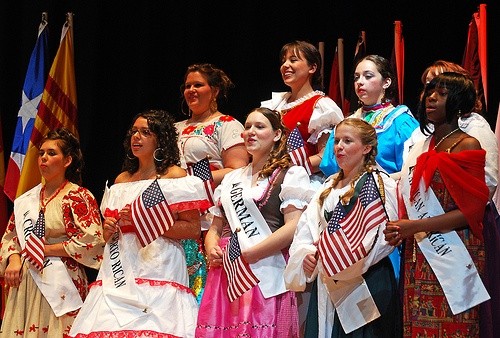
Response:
[129,129,154,137]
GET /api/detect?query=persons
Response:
[318,55,421,272]
[261,41,344,180]
[383,71,491,338]
[0,130,105,338]
[179,64,251,186]
[194,107,314,338]
[68,109,211,338]
[404,61,500,338]
[284,118,398,338]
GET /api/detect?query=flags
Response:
[20,210,45,274]
[131,179,174,248]
[318,202,366,277]
[339,173,387,252]
[287,128,314,176]
[3,20,50,203]
[222,229,261,303]
[186,158,217,215]
[16,20,82,196]
